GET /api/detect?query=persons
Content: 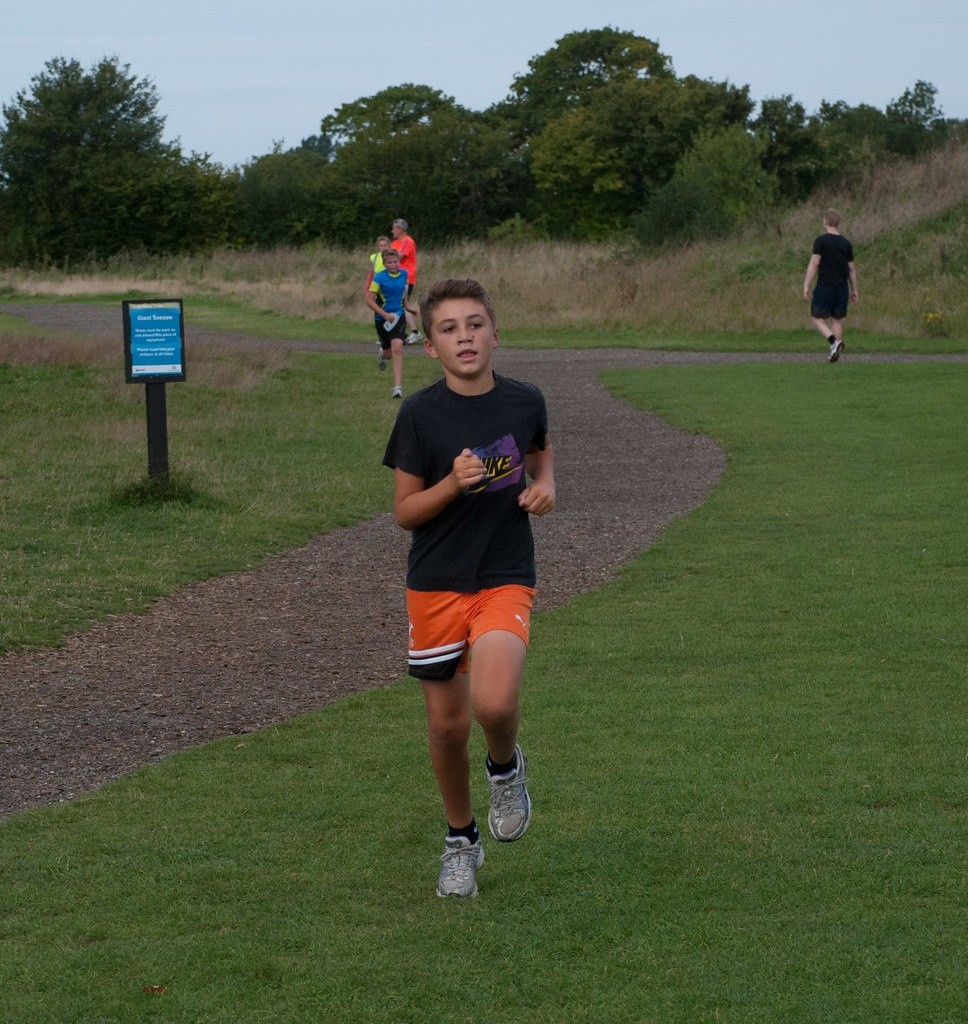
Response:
[803,209,859,364]
[384,219,424,344]
[371,236,393,278]
[383,278,557,896]
[367,248,420,398]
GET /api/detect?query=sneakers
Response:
[377,355,387,371]
[435,832,487,901]
[482,743,531,843]
[406,331,423,345]
[391,388,403,399]
[376,339,381,346]
[829,340,845,363]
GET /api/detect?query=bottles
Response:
[383,310,403,332]
